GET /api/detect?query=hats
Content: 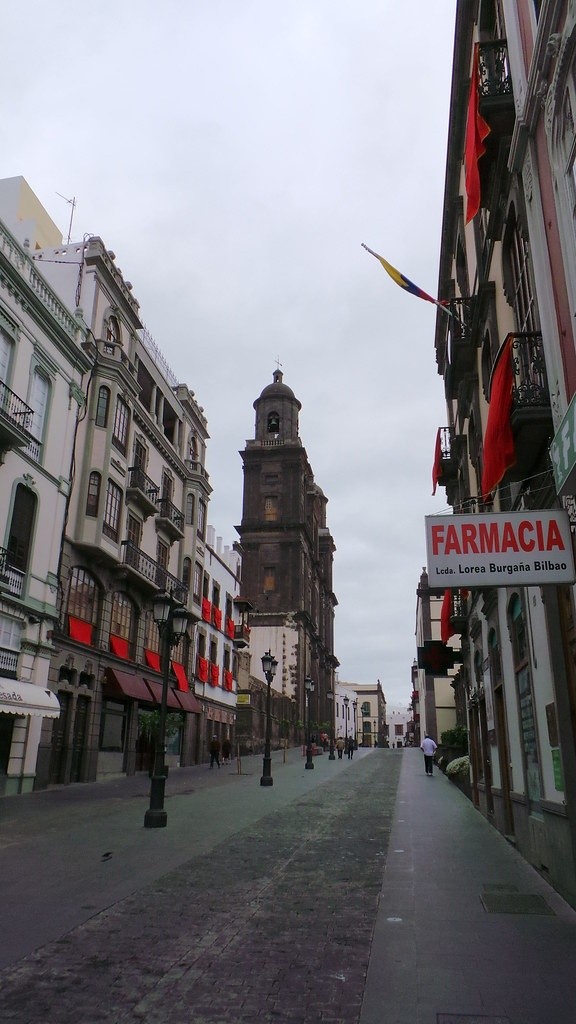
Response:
[212,735,217,738]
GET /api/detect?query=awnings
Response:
[145,678,181,708]
[109,667,154,702]
[173,687,204,715]
[0,675,61,719]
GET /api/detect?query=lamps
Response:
[28,615,41,624]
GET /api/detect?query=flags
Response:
[373,251,447,308]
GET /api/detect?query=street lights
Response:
[144,575,193,829]
[326,687,336,760]
[373,719,377,747]
[361,704,365,747]
[260,647,279,788]
[352,700,358,751]
[343,695,350,754]
[304,674,316,769]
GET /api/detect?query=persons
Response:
[420,734,438,775]
[335,737,345,759]
[346,736,354,760]
[322,739,328,751]
[222,738,232,765]
[208,737,221,768]
[311,736,315,743]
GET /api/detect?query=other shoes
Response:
[429,773,432,776]
[425,773,428,776]
[219,764,221,768]
[209,766,213,768]
